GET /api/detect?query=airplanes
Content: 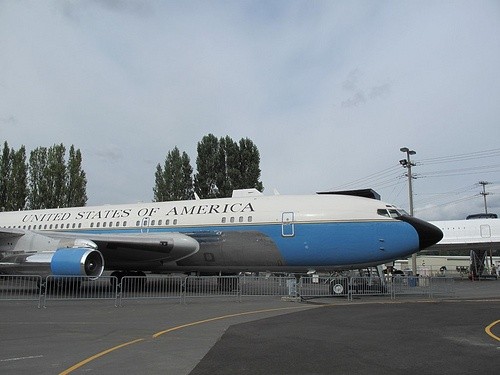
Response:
[0,189,444,296]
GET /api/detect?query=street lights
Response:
[399,147,417,274]
